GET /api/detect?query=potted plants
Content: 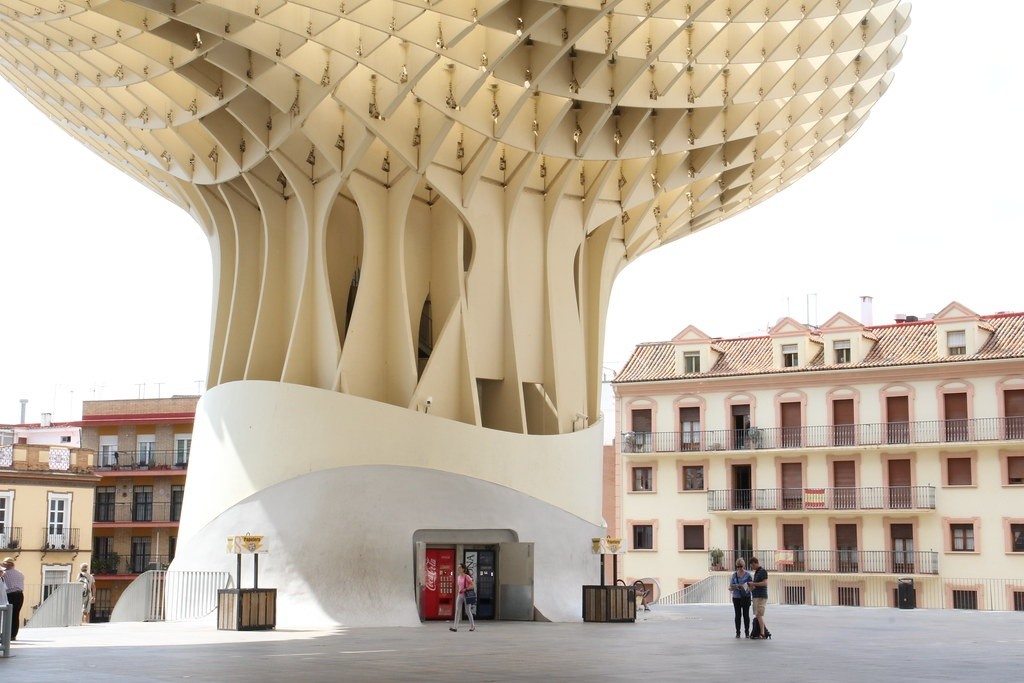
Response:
[710,550,724,571]
[91,553,121,574]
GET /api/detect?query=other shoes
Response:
[757,634,766,639]
[736,635,740,638]
[469,628,475,631]
[746,635,749,638]
[449,628,457,632]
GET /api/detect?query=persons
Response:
[729,557,752,638]
[77,563,96,623]
[2,557,25,641]
[747,557,768,639]
[449,564,476,632]
[0,566,9,645]
[636,582,650,611]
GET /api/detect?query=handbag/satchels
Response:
[740,593,751,607]
[464,574,477,604]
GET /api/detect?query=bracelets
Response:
[92,597,95,599]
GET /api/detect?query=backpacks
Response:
[750,618,772,639]
[78,572,89,597]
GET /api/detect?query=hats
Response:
[0,566,6,571]
[3,557,15,565]
